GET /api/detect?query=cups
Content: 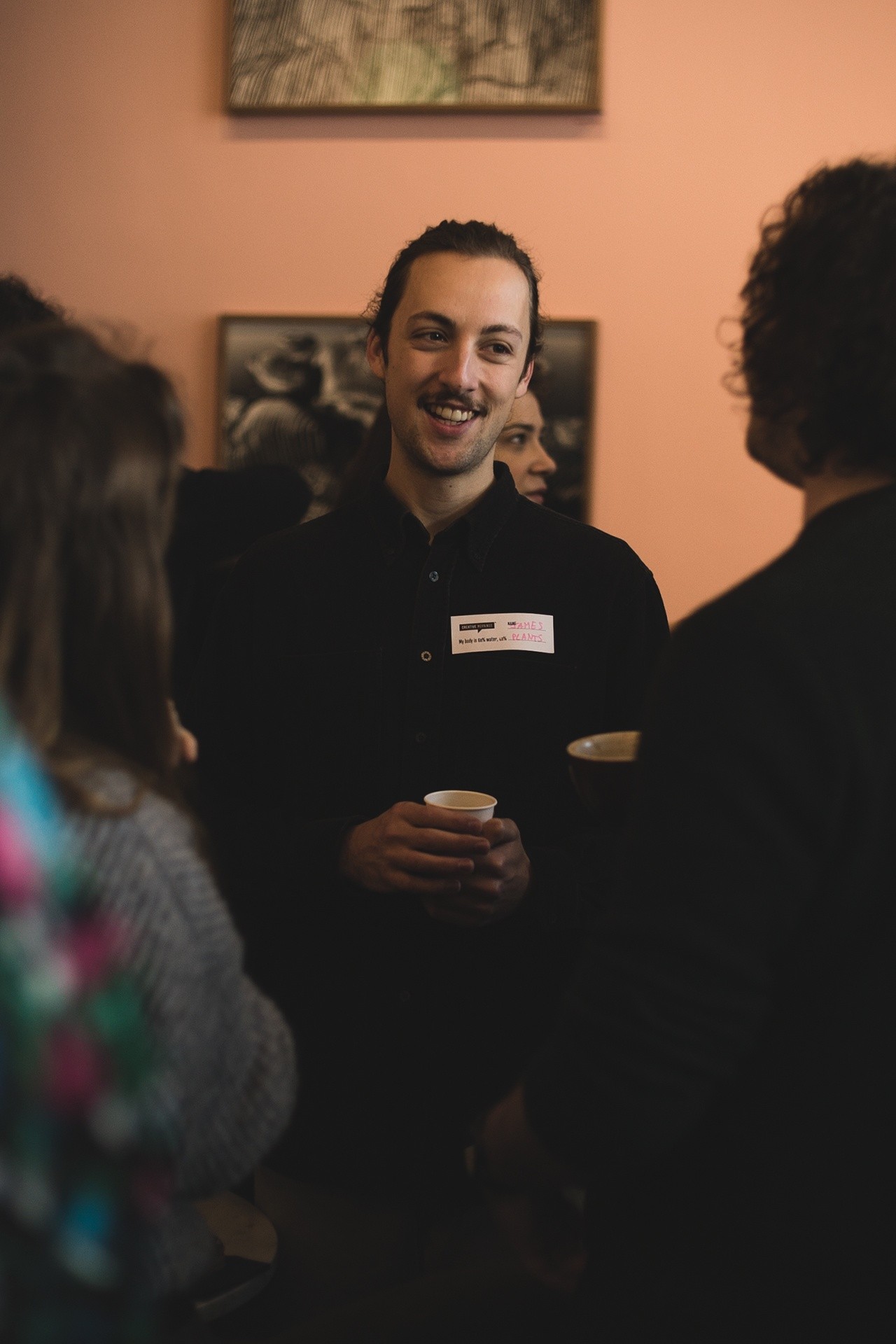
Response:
[423,790,497,824]
[567,729,642,828]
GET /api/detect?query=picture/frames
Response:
[214,315,599,526]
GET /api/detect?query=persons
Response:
[0,276,300,1344]
[532,154,896,1344]
[146,461,311,1033]
[0,706,176,1344]
[358,380,555,508]
[189,220,674,1344]
